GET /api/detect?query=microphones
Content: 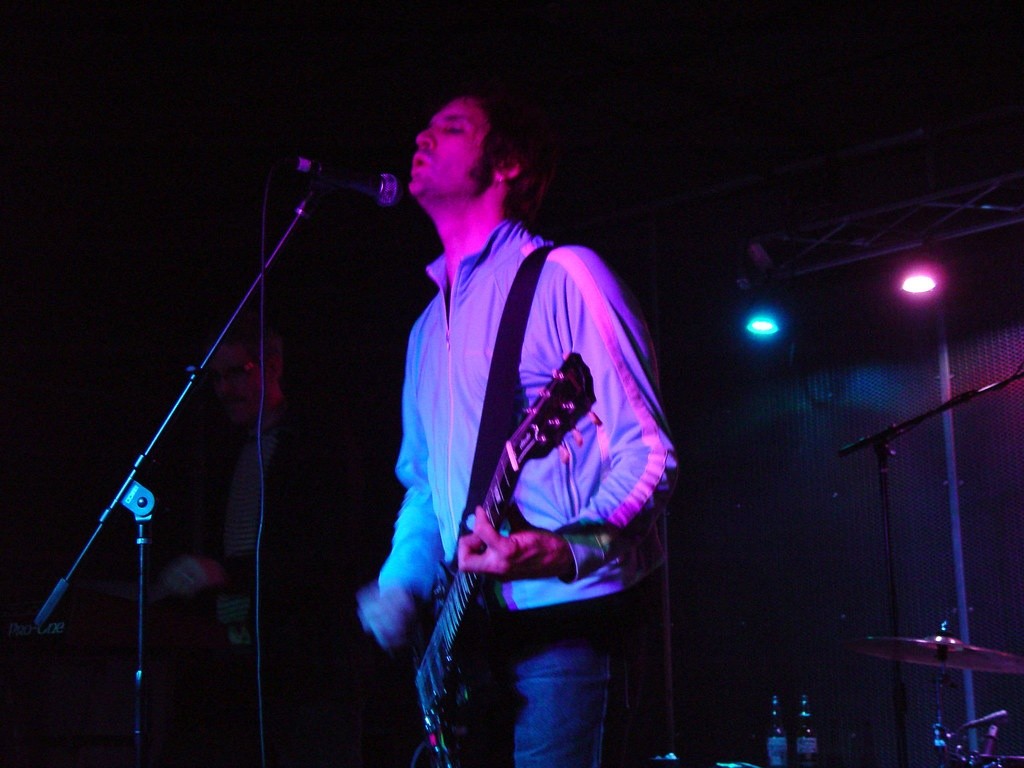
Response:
[290,156,402,206]
[960,709,1008,730]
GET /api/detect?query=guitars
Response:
[359,350,598,768]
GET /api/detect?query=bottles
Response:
[796,695,820,768]
[766,695,789,768]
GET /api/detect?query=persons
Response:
[165,306,358,768]
[354,80,683,768]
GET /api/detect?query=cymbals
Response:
[848,630,1024,674]
